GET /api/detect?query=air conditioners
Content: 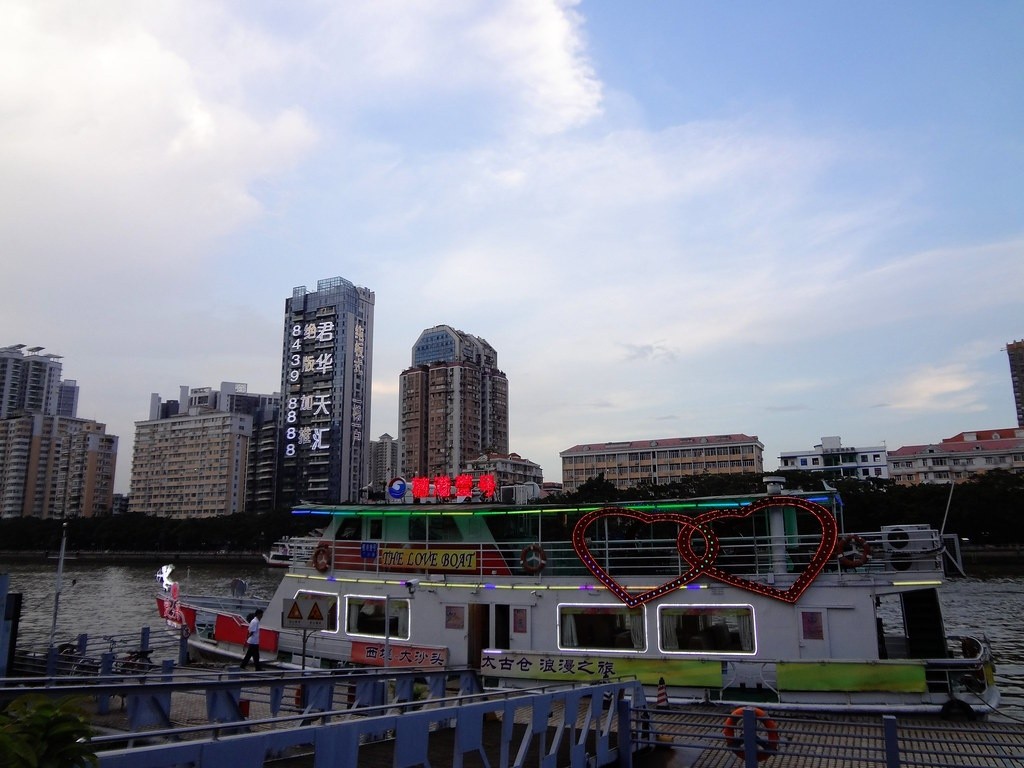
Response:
[881,524,938,572]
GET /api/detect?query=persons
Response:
[240,609,266,671]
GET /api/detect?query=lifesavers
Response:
[520,544,548,575]
[723,706,778,763]
[312,546,331,574]
[292,683,323,722]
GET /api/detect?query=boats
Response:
[48,551,79,560]
[154,475,1000,719]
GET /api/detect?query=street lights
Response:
[48,522,69,648]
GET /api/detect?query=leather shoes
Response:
[241,666,249,672]
[254,667,267,672]
